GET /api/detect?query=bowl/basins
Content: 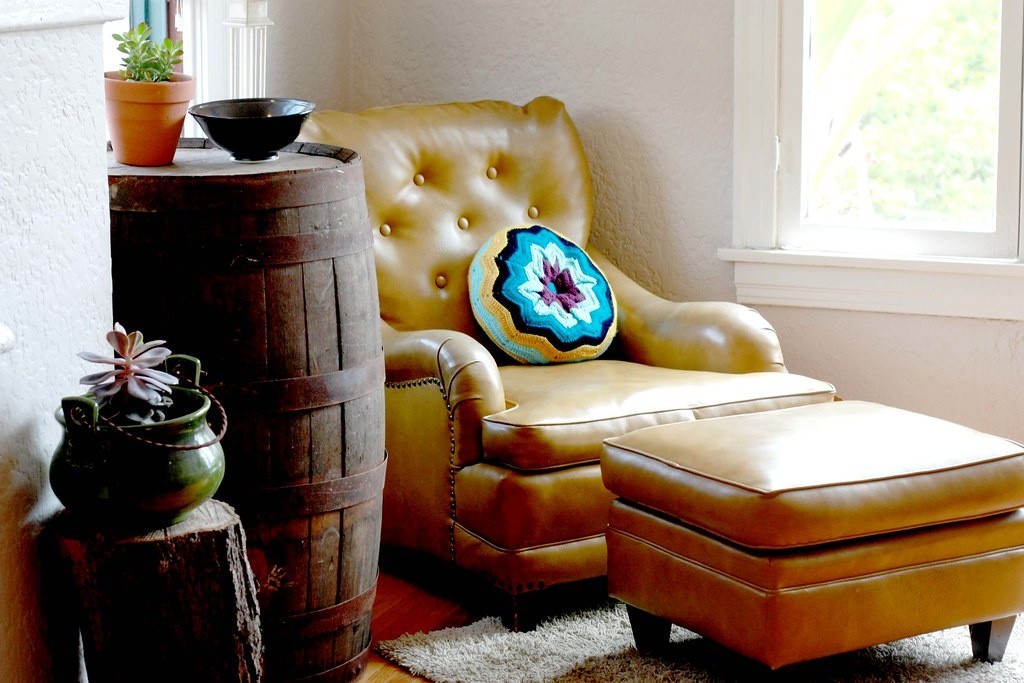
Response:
[188,98,317,160]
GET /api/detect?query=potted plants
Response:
[49,322,227,532]
[104,21,197,167]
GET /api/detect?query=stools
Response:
[602,401,1023,683]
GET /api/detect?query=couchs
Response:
[296,96,840,635]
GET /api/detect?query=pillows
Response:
[468,222,620,365]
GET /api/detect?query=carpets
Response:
[374,601,1023,683]
